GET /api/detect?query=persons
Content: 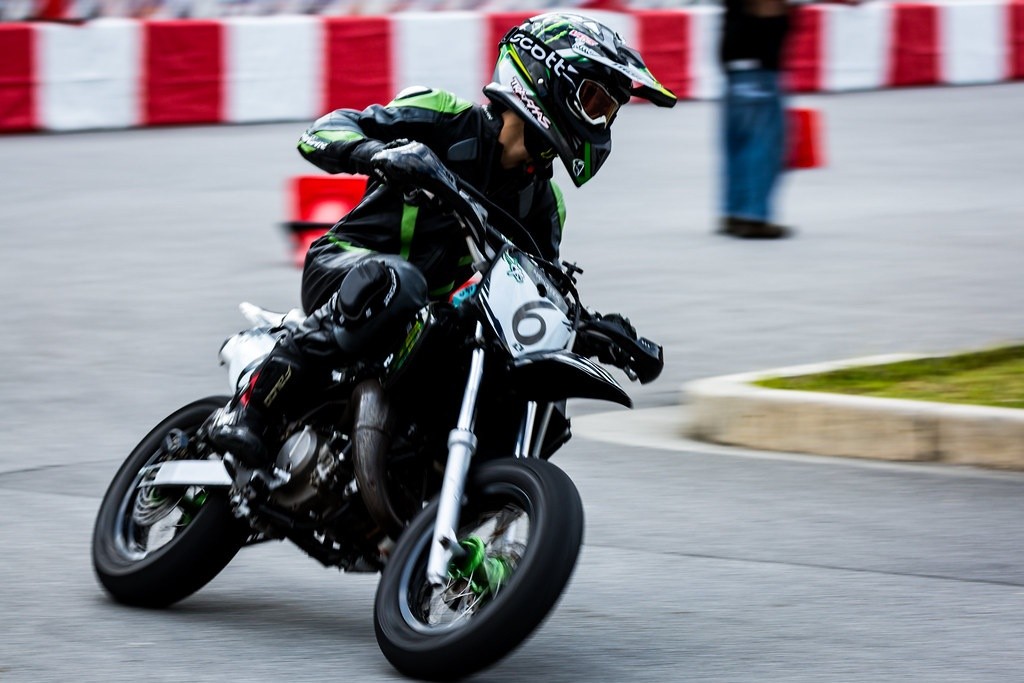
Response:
[204,15,676,462]
[715,0,866,239]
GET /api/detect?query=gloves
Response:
[348,137,438,196]
[583,309,641,373]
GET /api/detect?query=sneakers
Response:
[204,400,264,457]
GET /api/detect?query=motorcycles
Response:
[88,152,665,683]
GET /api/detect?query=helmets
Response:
[483,10,675,193]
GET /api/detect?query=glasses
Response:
[499,26,620,134]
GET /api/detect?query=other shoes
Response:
[721,215,785,238]
